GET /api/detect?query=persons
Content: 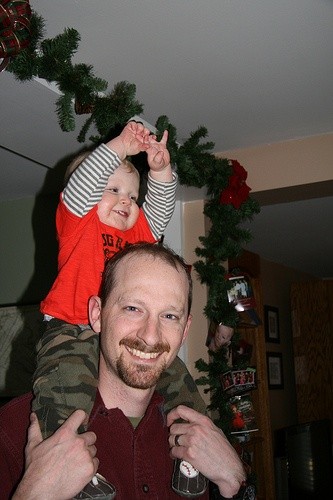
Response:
[208,325,260,390]
[0,240,259,500]
[29,120,208,499]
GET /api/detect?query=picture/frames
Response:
[264,305,281,344]
[266,351,284,389]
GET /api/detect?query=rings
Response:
[174,434,181,446]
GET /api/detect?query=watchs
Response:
[220,477,257,500]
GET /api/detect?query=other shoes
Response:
[172,460,208,496]
[78,474,116,499]
